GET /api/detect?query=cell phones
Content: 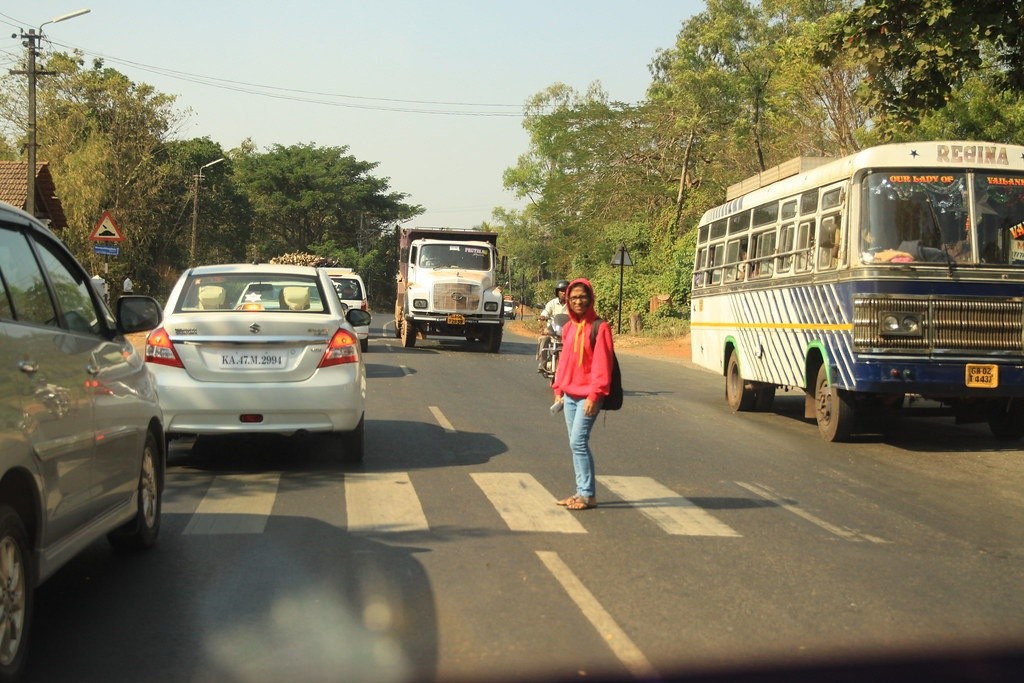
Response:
[550,398,563,416]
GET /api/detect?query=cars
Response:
[135,263,371,464]
[232,279,348,315]
[0,198,168,683]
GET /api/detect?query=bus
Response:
[690,137,1024,444]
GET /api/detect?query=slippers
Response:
[556,495,579,505]
[566,500,598,510]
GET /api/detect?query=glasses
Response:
[568,294,589,303]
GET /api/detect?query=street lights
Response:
[22,7,93,216]
[191,157,226,260]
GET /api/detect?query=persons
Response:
[551,278,613,511]
[538,281,569,374]
[695,226,990,288]
[123,273,134,295]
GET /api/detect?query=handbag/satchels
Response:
[590,318,623,410]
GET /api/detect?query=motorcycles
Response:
[535,300,571,389]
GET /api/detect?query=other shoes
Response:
[537,364,546,373]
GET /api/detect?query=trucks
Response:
[394,228,508,352]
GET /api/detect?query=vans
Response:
[504,299,516,319]
[311,266,370,352]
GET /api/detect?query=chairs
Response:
[280,286,311,311]
[342,286,353,299]
[199,286,227,309]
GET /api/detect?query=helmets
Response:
[555,281,570,297]
